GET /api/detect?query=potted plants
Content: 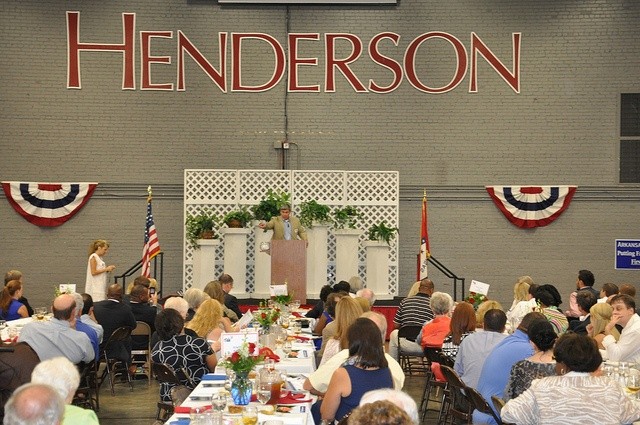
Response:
[220,210,256,229]
[184,212,218,251]
[252,189,289,233]
[331,205,362,230]
[365,223,401,248]
[299,198,333,233]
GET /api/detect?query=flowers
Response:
[466,289,488,312]
[215,337,270,405]
[272,290,294,306]
[249,303,281,339]
[54,286,73,300]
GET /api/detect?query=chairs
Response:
[70,361,98,414]
[148,362,187,425]
[95,324,135,397]
[437,349,460,425]
[463,383,502,425]
[439,363,475,425]
[1,340,42,421]
[489,394,518,425]
[396,322,433,383]
[129,320,156,391]
[417,345,446,425]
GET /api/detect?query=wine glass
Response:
[265,298,301,321]
[282,339,292,361]
[626,373,640,401]
[203,409,222,425]
[189,406,206,425]
[242,406,258,425]
[211,392,227,421]
[273,369,288,398]
[264,359,275,382]
[7,328,17,344]
[35,306,47,320]
[604,361,628,383]
[256,382,271,406]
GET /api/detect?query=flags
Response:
[142,184,161,278]
[417,188,431,282]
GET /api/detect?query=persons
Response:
[389,279,434,361]
[500,333,640,425]
[472,312,548,425]
[81,294,104,345]
[18,294,96,366]
[356,288,376,313]
[3,382,65,425]
[310,317,394,425]
[475,300,502,329]
[150,296,198,351]
[313,285,336,351]
[508,275,539,326]
[302,311,406,399]
[589,303,621,349]
[219,273,243,323]
[597,282,619,304]
[594,294,640,362]
[1,280,29,321]
[30,355,100,425]
[359,388,420,424]
[535,284,569,333]
[620,284,636,297]
[258,203,309,249]
[441,302,477,356]
[327,292,340,308]
[134,276,150,289]
[607,294,618,303]
[184,288,205,310]
[93,284,137,362]
[148,279,157,299]
[150,309,218,419]
[203,281,238,323]
[417,292,453,383]
[331,281,350,293]
[84,239,115,302]
[187,299,231,352]
[125,285,156,350]
[338,399,417,425]
[570,270,600,315]
[503,321,575,400]
[354,297,370,313]
[318,296,363,370]
[67,292,100,365]
[5,270,34,317]
[575,290,593,334]
[453,309,508,413]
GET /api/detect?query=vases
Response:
[228,367,255,407]
[257,325,277,351]
[278,303,290,318]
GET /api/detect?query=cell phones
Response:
[150,287,155,297]
